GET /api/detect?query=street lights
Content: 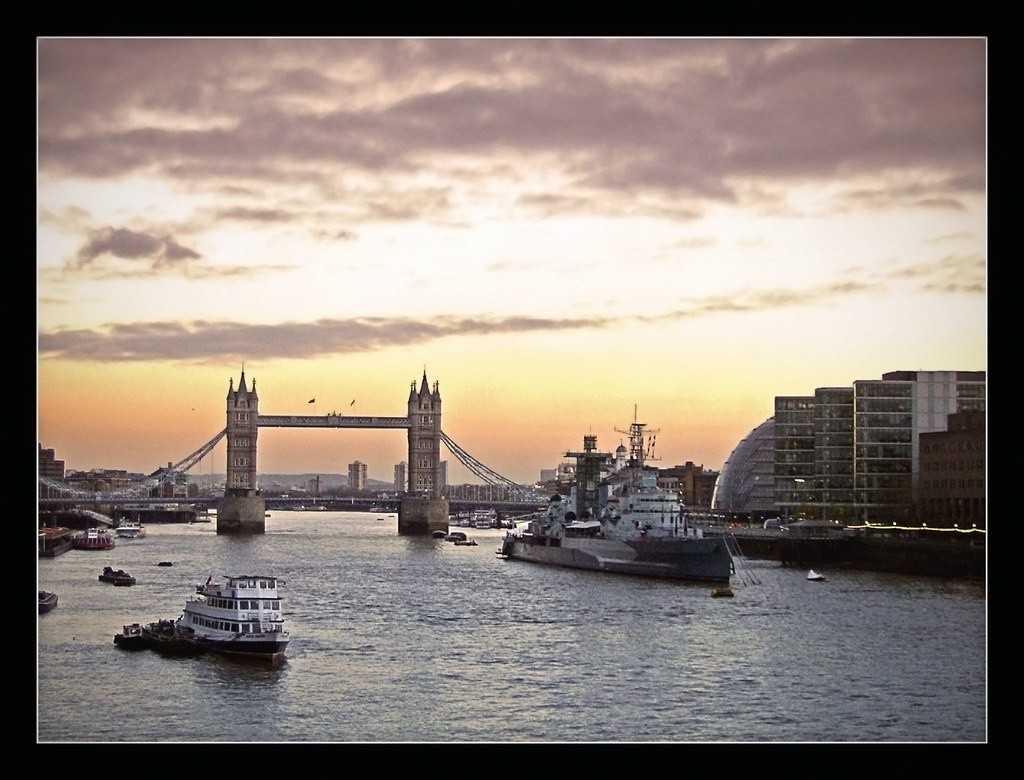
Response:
[37,588,58,614]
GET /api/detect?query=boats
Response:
[432,529,479,546]
[115,515,146,539]
[492,402,736,583]
[806,568,823,583]
[71,527,116,551]
[176,574,291,659]
[99,565,136,586]
[113,618,215,653]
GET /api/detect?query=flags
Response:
[307,399,315,403]
[351,400,355,406]
